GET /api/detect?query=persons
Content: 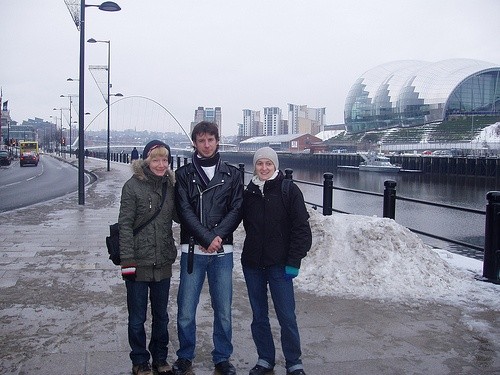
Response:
[243,145,315,375]
[85,149,89,158]
[174,122,243,375]
[131,147,139,163]
[120,140,181,375]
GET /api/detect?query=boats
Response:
[358,143,403,174]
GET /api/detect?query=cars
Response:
[422,150,450,155]
[19,152,39,166]
[0,150,12,166]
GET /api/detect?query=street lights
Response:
[86,37,123,172]
[77,0,121,205]
[50,78,90,158]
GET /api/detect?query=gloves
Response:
[122,265,137,283]
[283,265,300,279]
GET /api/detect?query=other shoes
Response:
[170,358,192,375]
[215,361,236,375]
[249,364,274,375]
[152,359,175,375]
[286,369,306,375]
[131,361,153,375]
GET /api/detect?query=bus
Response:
[20,141,40,162]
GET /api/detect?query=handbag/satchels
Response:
[105,223,121,266]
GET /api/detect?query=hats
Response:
[254,147,279,171]
[143,140,172,165]
